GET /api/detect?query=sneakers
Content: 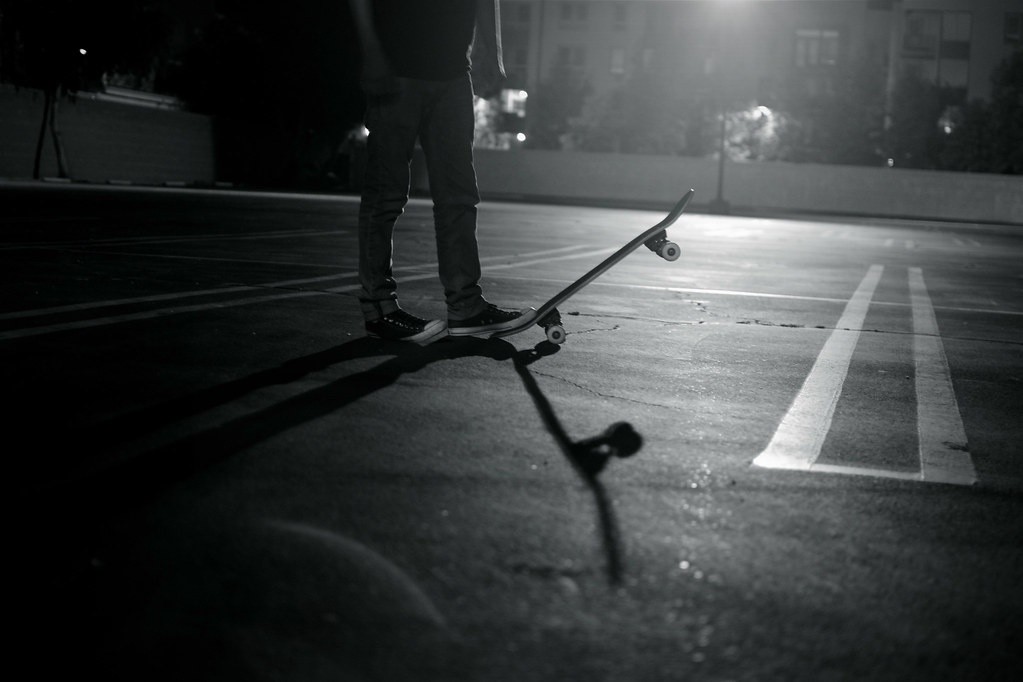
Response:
[365,308,448,346]
[447,303,537,336]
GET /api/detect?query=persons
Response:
[352,0,539,339]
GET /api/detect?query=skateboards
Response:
[489,185,696,345]
